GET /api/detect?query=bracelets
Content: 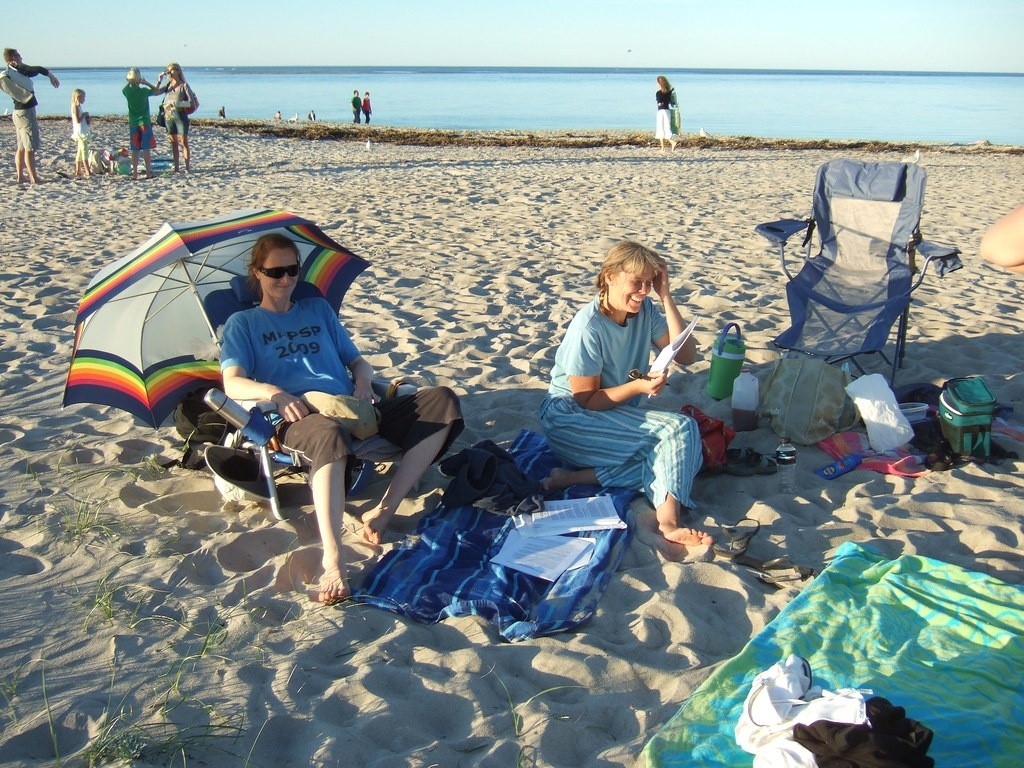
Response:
[156,80,162,84]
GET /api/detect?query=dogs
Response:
[96,147,128,175]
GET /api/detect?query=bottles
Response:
[775,438,796,493]
[732,368,760,432]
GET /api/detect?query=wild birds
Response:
[366,135,373,152]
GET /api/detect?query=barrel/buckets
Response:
[706,323,746,401]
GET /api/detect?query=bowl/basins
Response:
[899,402,929,421]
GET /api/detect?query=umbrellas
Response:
[61,207,376,433]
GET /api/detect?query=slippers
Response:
[713,518,760,558]
[726,447,779,476]
[736,555,818,593]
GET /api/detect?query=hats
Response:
[204,445,271,504]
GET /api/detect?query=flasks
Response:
[204,389,251,429]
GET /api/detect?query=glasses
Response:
[257,260,300,279]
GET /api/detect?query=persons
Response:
[71,89,94,176]
[122,68,160,179]
[219,233,467,603]
[540,241,716,546]
[308,110,315,121]
[155,63,195,175]
[3,48,60,183]
[351,90,372,124]
[274,110,282,120]
[655,76,677,152]
[218,106,226,118]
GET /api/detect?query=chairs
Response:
[204,277,420,521]
[753,156,962,390]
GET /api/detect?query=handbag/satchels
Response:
[681,405,735,475]
[89,149,105,174]
[0,63,34,104]
[156,105,166,127]
[162,390,237,472]
[183,82,200,115]
[938,377,996,458]
[761,351,860,446]
